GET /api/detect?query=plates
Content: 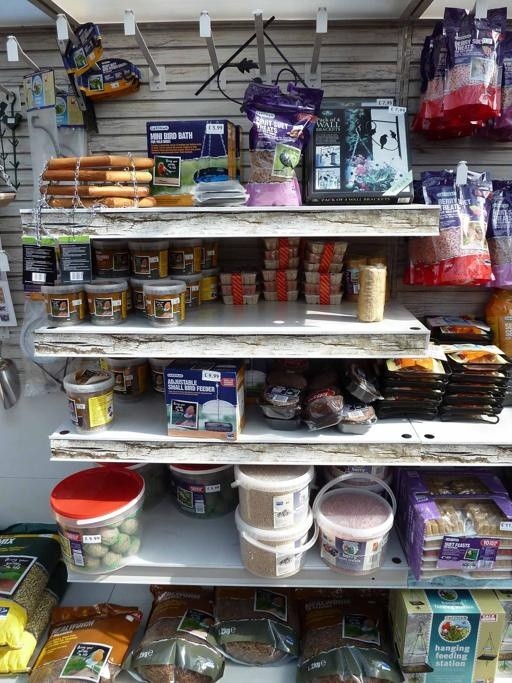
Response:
[32,76,42,97]
[342,544,358,555]
[437,590,458,601]
[55,97,66,115]
[438,619,471,644]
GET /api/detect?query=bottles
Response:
[41,238,220,329]
[63,357,174,435]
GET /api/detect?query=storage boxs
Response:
[494,590,512,677]
[390,591,507,683]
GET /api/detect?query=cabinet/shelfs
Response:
[19,204,512,590]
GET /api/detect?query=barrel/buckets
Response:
[170,462,240,520]
[53,469,147,576]
[230,466,314,529]
[313,473,397,576]
[233,506,318,579]
[98,457,166,504]
